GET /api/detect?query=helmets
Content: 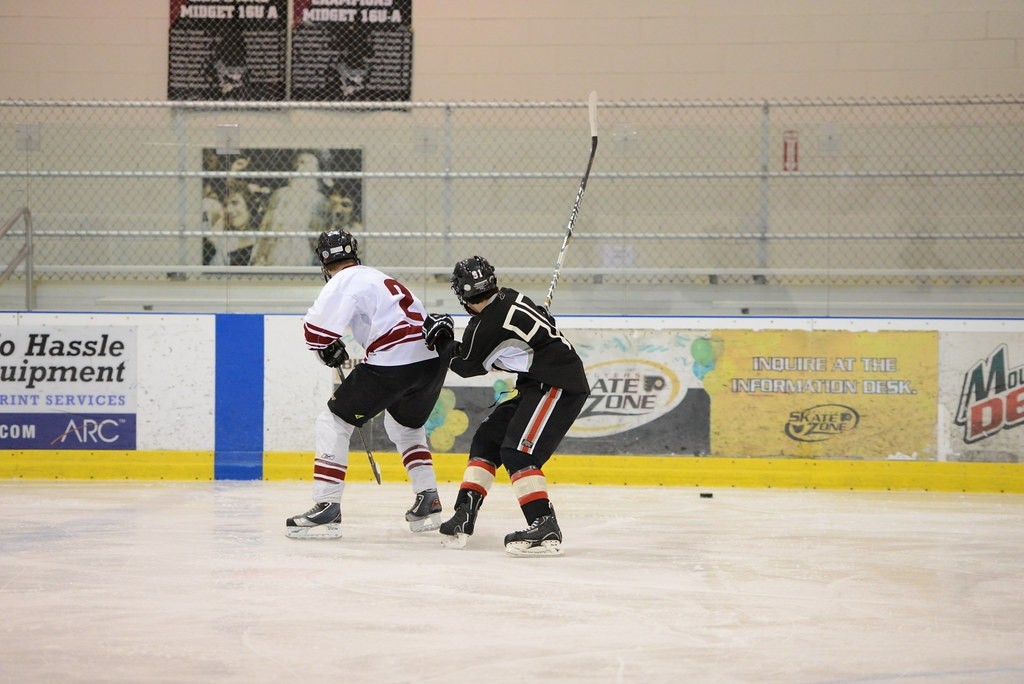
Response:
[315,227,358,265]
[450,256,497,304]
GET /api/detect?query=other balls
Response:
[700,492,713,498]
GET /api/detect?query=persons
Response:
[203,149,362,265]
[285,229,449,541]
[422,255,592,555]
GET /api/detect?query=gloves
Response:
[317,339,349,368]
[422,312,455,351]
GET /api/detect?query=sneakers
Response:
[439,488,484,550]
[504,498,563,556]
[404,488,443,533]
[285,502,342,539]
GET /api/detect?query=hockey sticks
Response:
[543,92,600,310]
[329,344,382,485]
